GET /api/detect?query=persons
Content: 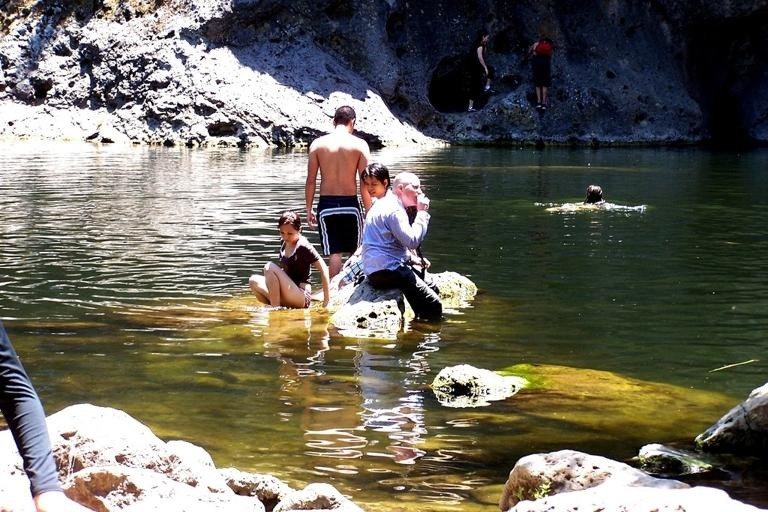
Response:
[466,30,496,113]
[0,318,69,512]
[248,209,331,312]
[361,170,444,322]
[336,160,390,291]
[527,38,554,110]
[583,184,606,206]
[304,104,373,280]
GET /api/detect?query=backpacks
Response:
[535,39,552,56]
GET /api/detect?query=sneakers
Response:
[541,104,547,109]
[535,103,542,108]
[483,88,495,94]
[468,106,477,112]
